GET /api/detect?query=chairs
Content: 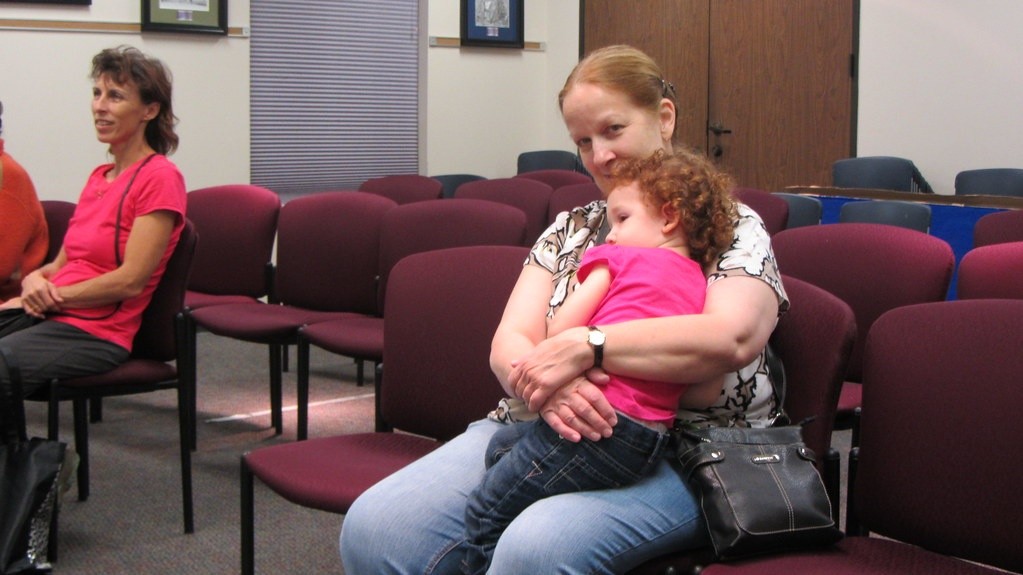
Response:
[0,155,1023,575]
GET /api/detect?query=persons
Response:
[339,43,790,575]
[1,46,187,486]
[458,145,736,575]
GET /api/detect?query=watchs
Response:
[587,324,606,369]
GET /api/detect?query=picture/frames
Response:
[141,0,228,34]
[459,0,524,48]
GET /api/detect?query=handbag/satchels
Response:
[1,437,78,575]
[674,419,840,558]
